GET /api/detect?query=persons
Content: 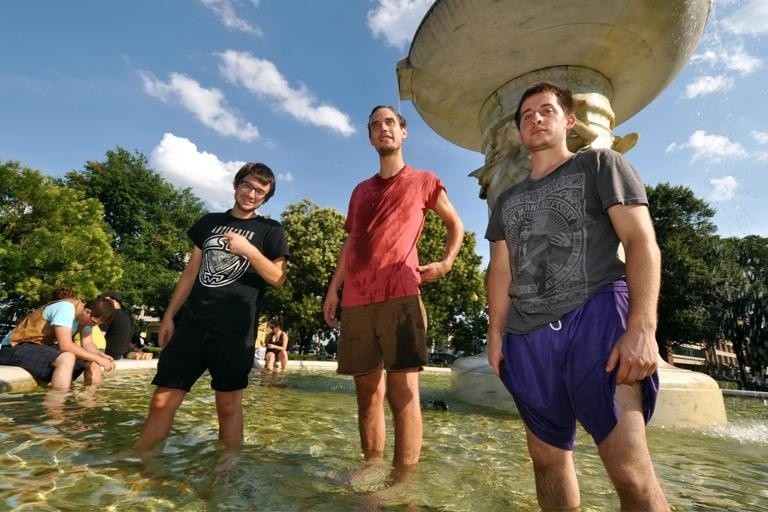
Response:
[95,292,132,361]
[322,104,465,507]
[483,81,672,512]
[133,162,291,452]
[0,296,116,393]
[263,320,290,373]
[51,285,106,355]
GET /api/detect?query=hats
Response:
[97,291,123,302]
